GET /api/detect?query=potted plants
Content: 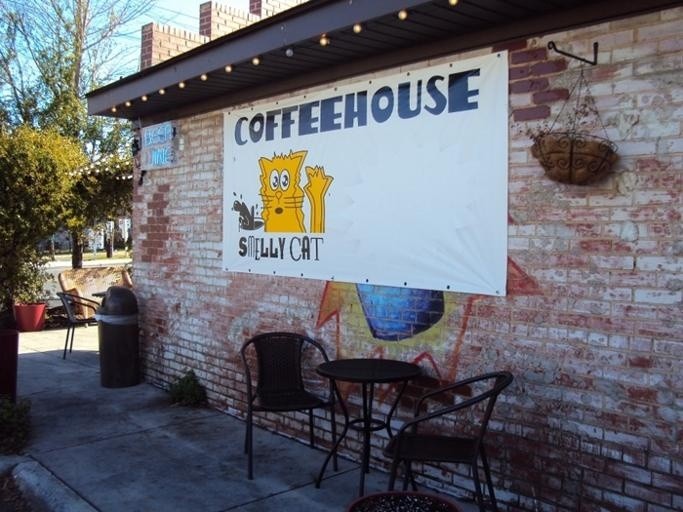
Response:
[13,247,45,332]
[511,59,618,185]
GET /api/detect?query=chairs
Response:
[383,371,513,512]
[56,293,100,360]
[240,332,338,480]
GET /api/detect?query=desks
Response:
[315,358,422,498]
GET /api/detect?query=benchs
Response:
[58,266,133,328]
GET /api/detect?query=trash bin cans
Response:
[94,286,141,387]
